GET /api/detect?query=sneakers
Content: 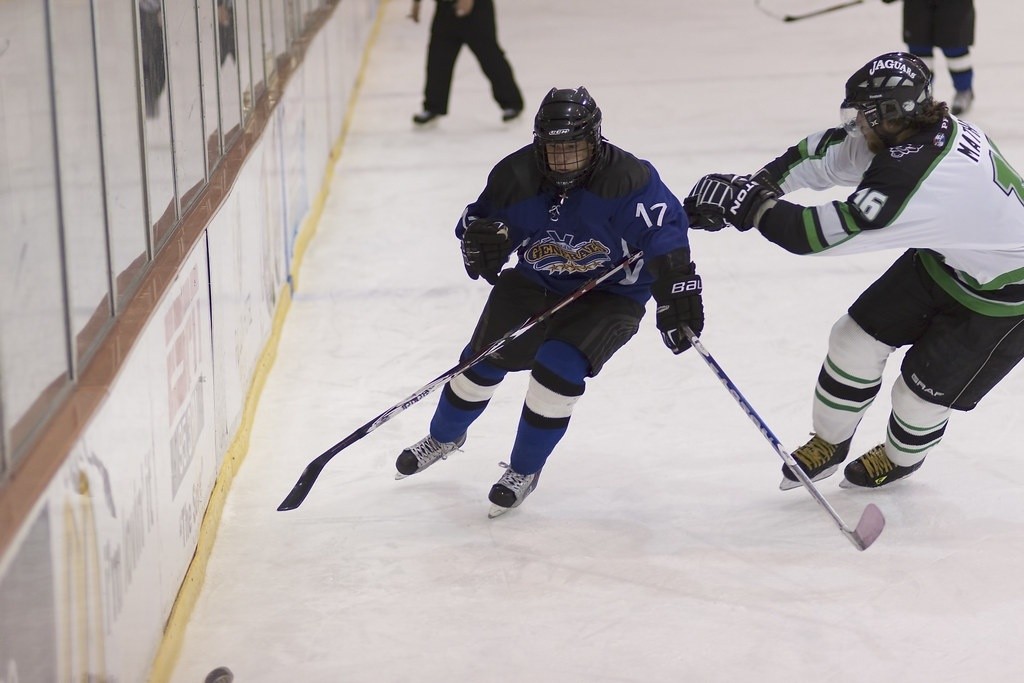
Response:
[840,442,926,490]
[488,460,542,519]
[395,430,468,481]
[779,431,856,489]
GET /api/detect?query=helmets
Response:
[533,85,602,192]
[840,53,933,136]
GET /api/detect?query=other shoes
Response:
[952,89,975,117]
[501,106,523,121]
[412,110,437,124]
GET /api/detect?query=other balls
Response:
[204,665,234,683]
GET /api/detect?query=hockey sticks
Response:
[680,320,890,554]
[755,1,866,25]
[274,213,701,513]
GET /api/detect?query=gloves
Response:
[682,167,784,232]
[648,261,704,355]
[460,218,511,285]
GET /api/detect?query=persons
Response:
[903,0,975,116]
[685,53,1024,492]
[394,86,703,518]
[410,0,524,123]
